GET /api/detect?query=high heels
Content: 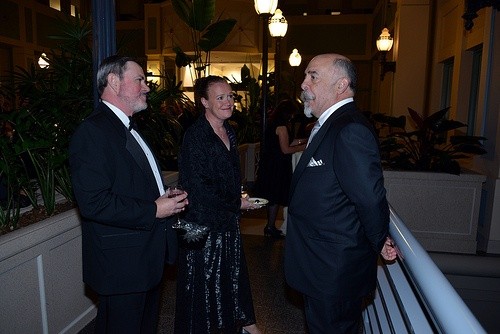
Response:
[263,223,282,237]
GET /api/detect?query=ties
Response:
[305,121,320,149]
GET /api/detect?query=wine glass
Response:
[168,185,187,228]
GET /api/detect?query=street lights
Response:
[268,7,288,145]
[254,0,280,144]
[288,47,301,148]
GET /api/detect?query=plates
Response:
[248,198,269,205]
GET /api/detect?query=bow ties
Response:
[128,115,136,131]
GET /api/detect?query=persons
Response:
[284,54,397,334]
[171,75,262,334]
[68,56,189,334]
[254,99,307,240]
[297,114,318,139]
